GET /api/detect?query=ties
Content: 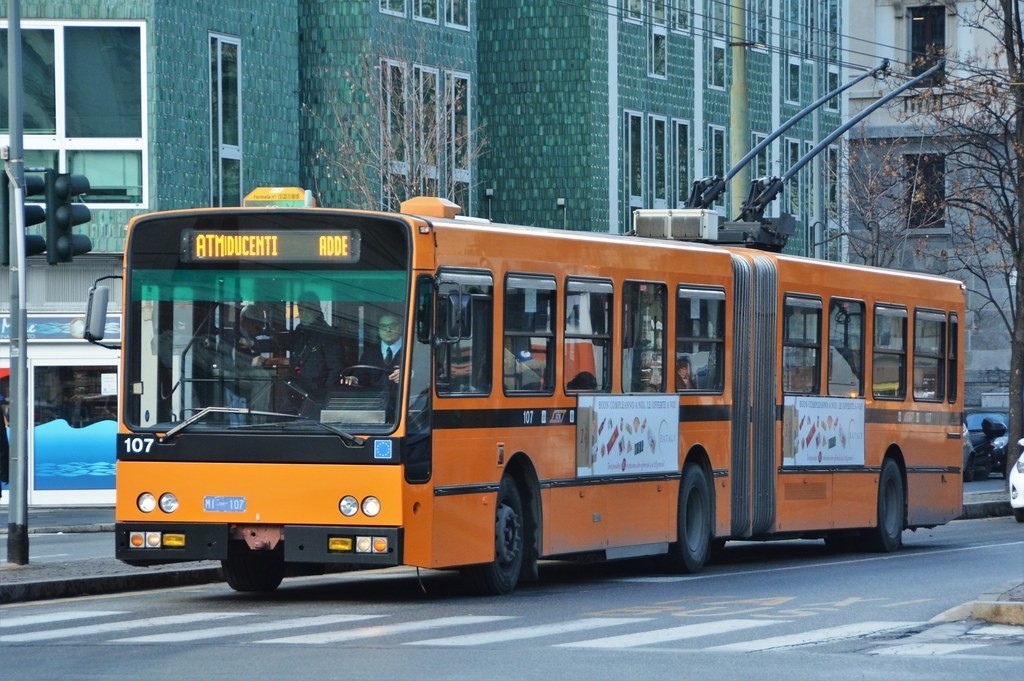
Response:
[385,347,393,365]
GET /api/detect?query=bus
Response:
[82,55,968,598]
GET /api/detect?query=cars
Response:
[962,407,1010,483]
[1008,437,1024,524]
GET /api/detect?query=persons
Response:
[676,355,694,391]
[343,314,427,406]
[238,291,344,421]
[215,305,265,425]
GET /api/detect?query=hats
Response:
[241,305,265,322]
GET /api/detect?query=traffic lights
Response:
[0,168,47,267]
[44,171,92,266]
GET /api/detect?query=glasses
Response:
[379,322,399,331]
